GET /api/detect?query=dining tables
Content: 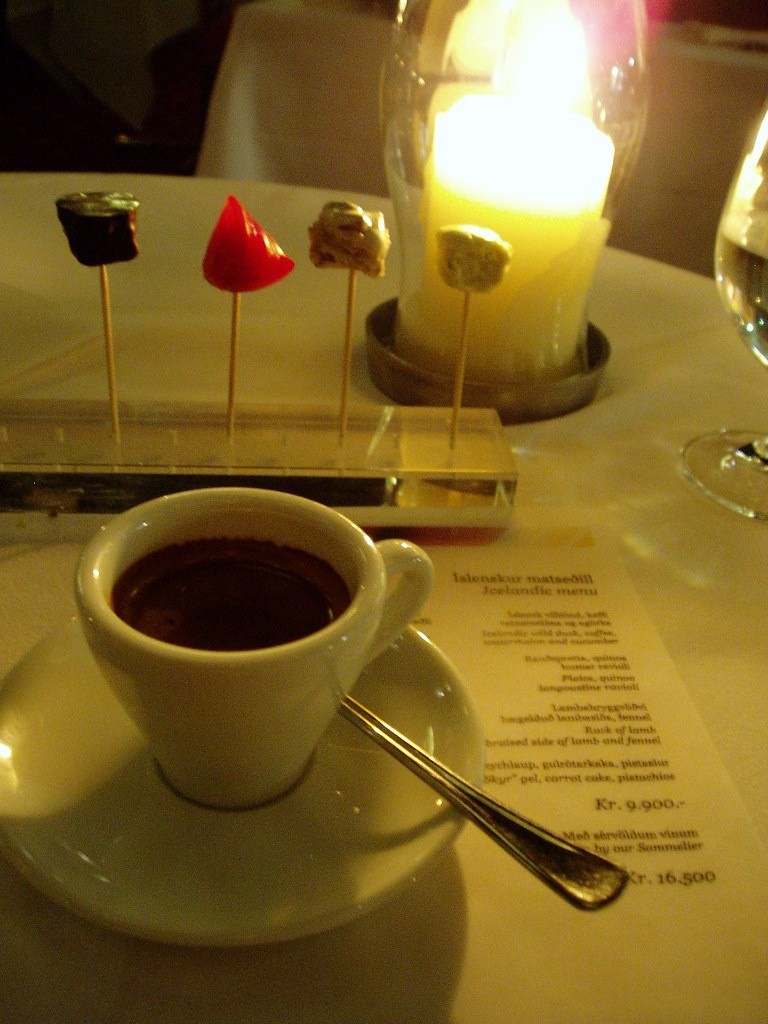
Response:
[0,166,767,1024]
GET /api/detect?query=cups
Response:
[73,485,435,809]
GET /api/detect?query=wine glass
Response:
[679,102,768,524]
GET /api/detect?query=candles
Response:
[416,0,616,382]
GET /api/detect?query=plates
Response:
[1,610,487,949]
